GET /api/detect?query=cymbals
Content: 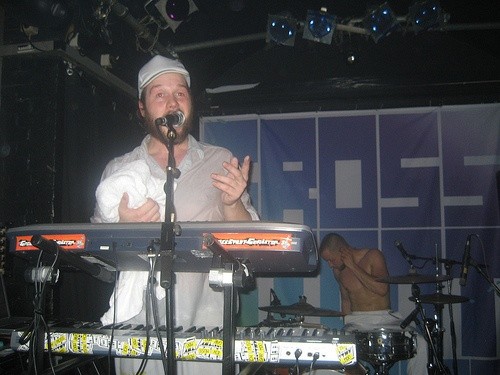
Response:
[373,274,454,284]
[258,302,346,316]
[407,294,468,305]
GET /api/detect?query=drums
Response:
[257,319,328,329]
[353,328,418,362]
[236,359,369,375]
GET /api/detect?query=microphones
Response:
[394,240,414,268]
[154,110,186,127]
[270,289,286,318]
[460,239,471,286]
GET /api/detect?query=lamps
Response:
[263,0,452,50]
[143,0,200,42]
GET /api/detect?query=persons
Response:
[318,233,430,375]
[90,55,260,375]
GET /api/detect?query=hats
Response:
[137,54,191,99]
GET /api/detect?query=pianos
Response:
[0,221,368,375]
